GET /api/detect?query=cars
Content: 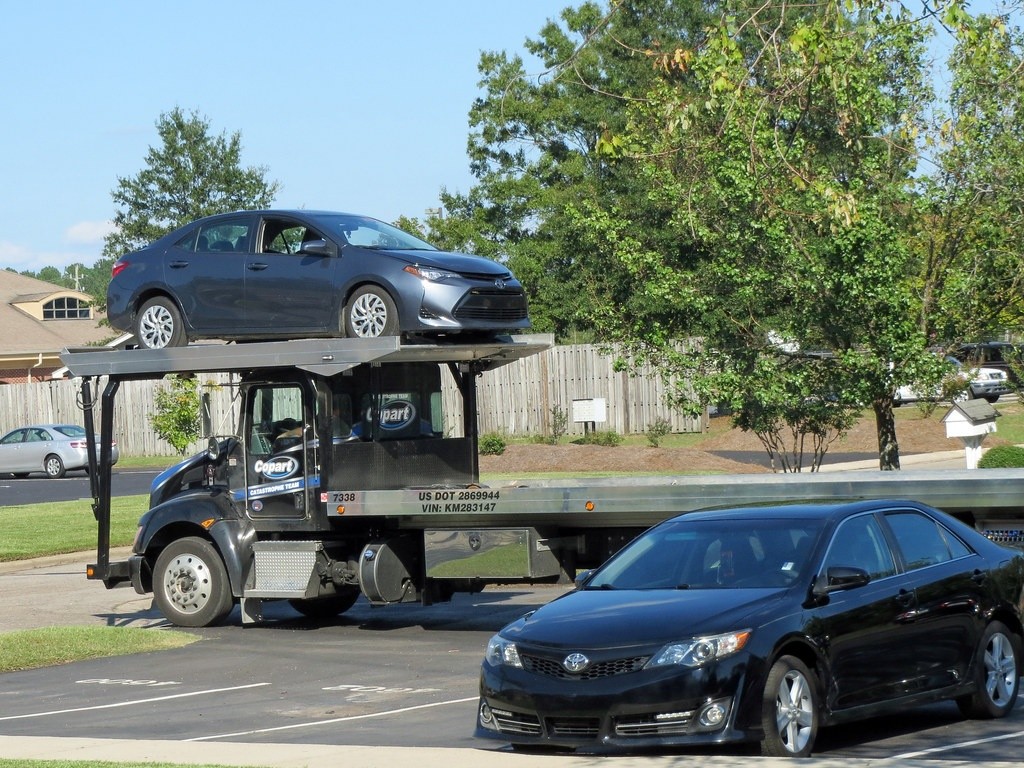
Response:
[885,356,1012,403]
[105,209,532,344]
[954,341,1024,394]
[0,424,119,479]
[476,502,1024,758]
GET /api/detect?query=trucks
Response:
[57,336,1023,629]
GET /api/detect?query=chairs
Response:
[275,426,302,449]
